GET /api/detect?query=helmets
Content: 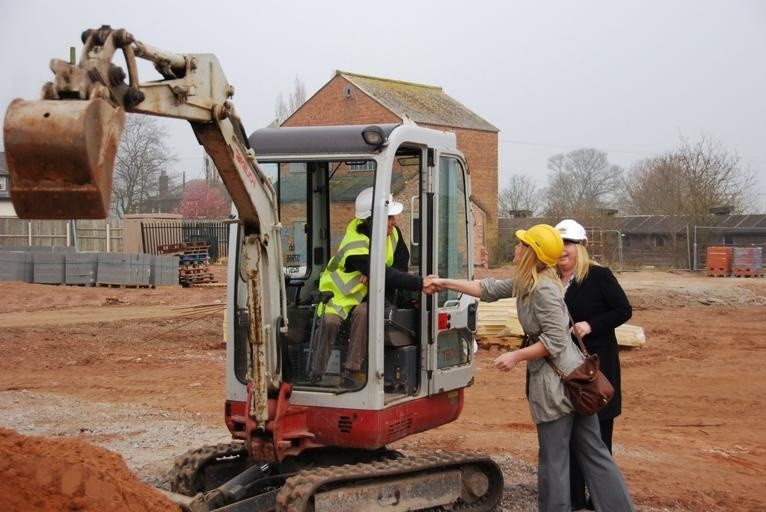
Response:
[355,186,403,219]
[515,219,587,268]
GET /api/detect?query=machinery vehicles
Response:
[2,23,506,510]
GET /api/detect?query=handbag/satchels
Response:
[563,354,615,417]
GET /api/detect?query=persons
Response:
[306,186,444,388]
